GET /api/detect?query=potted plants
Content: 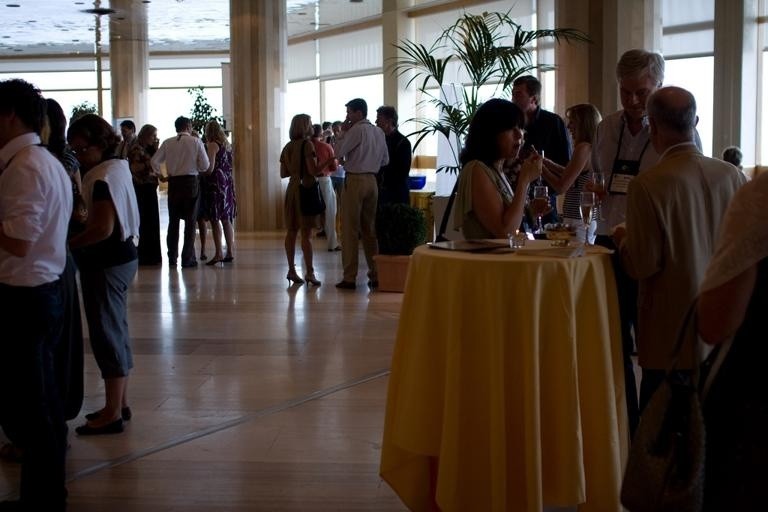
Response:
[371,202,433,295]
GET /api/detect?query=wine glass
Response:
[533,172,606,247]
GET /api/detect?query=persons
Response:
[190,129,211,261]
[612,87,745,418]
[127,125,164,265]
[693,172,768,512]
[721,145,743,174]
[279,98,412,289]
[0,79,73,511]
[67,113,140,435]
[150,116,210,267]
[196,120,236,268]
[582,48,663,443]
[115,120,136,158]
[4,99,84,463]
[460,100,542,253]
[512,76,571,239]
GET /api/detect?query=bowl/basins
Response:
[551,241,582,257]
[408,175,426,189]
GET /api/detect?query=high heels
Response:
[286,271,302,288]
[304,273,323,288]
[205,255,235,267]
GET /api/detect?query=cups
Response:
[508,232,526,248]
[535,150,544,159]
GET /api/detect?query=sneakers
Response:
[74,407,133,437]
[366,275,379,294]
[169,255,208,268]
[334,276,357,292]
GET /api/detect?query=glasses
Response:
[70,142,98,155]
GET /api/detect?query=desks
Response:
[329,173,362,248]
[430,194,451,237]
[409,188,437,243]
[377,240,632,511]
[408,176,426,189]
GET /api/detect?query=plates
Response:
[427,239,511,252]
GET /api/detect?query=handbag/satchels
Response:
[297,180,325,217]
